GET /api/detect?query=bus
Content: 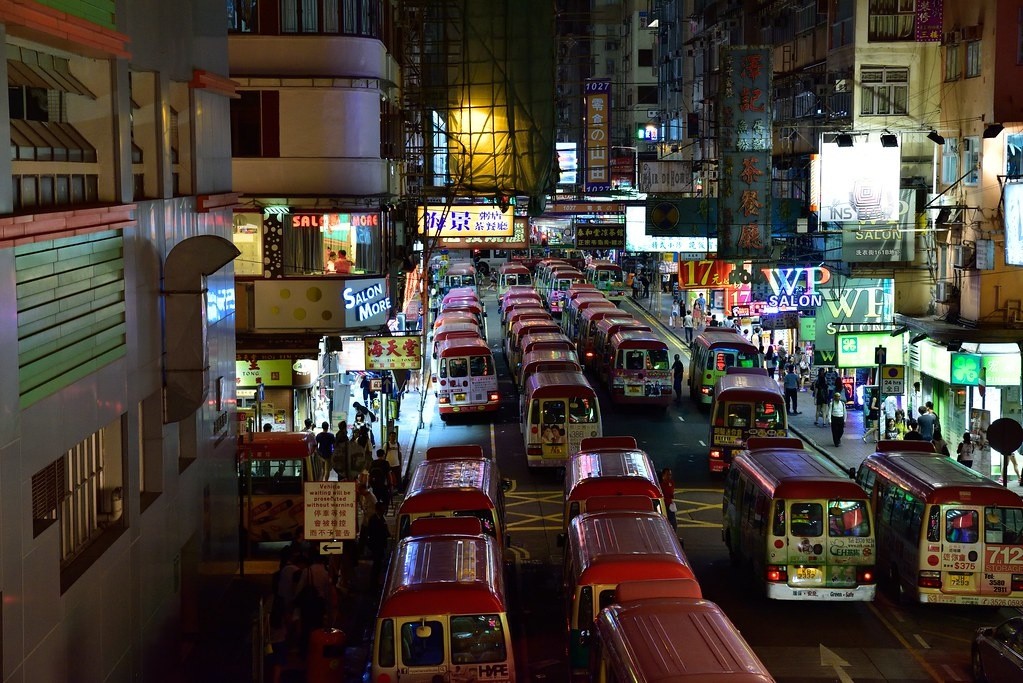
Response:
[722,437,876,619]
[559,435,668,532]
[370,515,517,683]
[848,438,1023,615]
[394,445,516,555]
[556,494,704,683]
[516,363,603,475]
[591,578,775,683]
[431,248,673,422]
[708,366,788,473]
[236,430,326,555]
[686,326,762,413]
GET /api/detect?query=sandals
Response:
[814,423,819,426]
[823,424,826,427]
[862,436,867,443]
[874,440,878,443]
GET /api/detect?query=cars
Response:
[970,615,1023,683]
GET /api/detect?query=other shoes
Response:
[398,491,403,496]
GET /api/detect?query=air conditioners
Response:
[947,31,961,45]
[726,19,741,30]
[721,21,726,31]
[700,41,708,49]
[620,25,626,37]
[622,60,630,72]
[715,30,721,39]
[686,49,696,57]
[814,84,834,97]
[941,32,952,46]
[935,281,953,304]
[662,49,682,64]
[961,24,982,41]
[953,245,971,269]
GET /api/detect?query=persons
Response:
[784,363,802,416]
[631,272,649,299]
[655,468,678,535]
[765,340,787,381]
[487,271,497,292]
[541,425,567,443]
[672,354,683,400]
[301,418,394,555]
[812,367,841,426]
[862,387,881,444]
[256,423,272,476]
[383,432,404,492]
[794,346,810,392]
[956,432,975,469]
[352,401,375,448]
[670,293,705,346]
[884,402,941,441]
[742,328,751,342]
[829,393,847,447]
[324,246,352,275]
[705,305,741,335]
[750,327,764,370]
[583,398,594,422]
[360,374,375,411]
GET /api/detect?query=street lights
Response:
[612,144,639,192]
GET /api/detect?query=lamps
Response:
[383,204,391,213]
[402,260,417,274]
[880,129,899,148]
[325,335,344,354]
[388,203,397,210]
[946,338,964,352]
[935,208,952,224]
[908,331,929,345]
[889,326,908,338]
[926,126,946,145]
[915,189,927,214]
[615,185,619,190]
[835,130,854,148]
[981,123,1005,138]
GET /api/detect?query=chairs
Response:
[451,632,476,651]
[453,652,477,663]
[273,463,285,483]
[478,631,503,644]
[456,366,467,377]
[471,359,493,376]
[479,618,502,633]
[453,620,475,633]
[260,462,269,477]
[477,648,503,663]
